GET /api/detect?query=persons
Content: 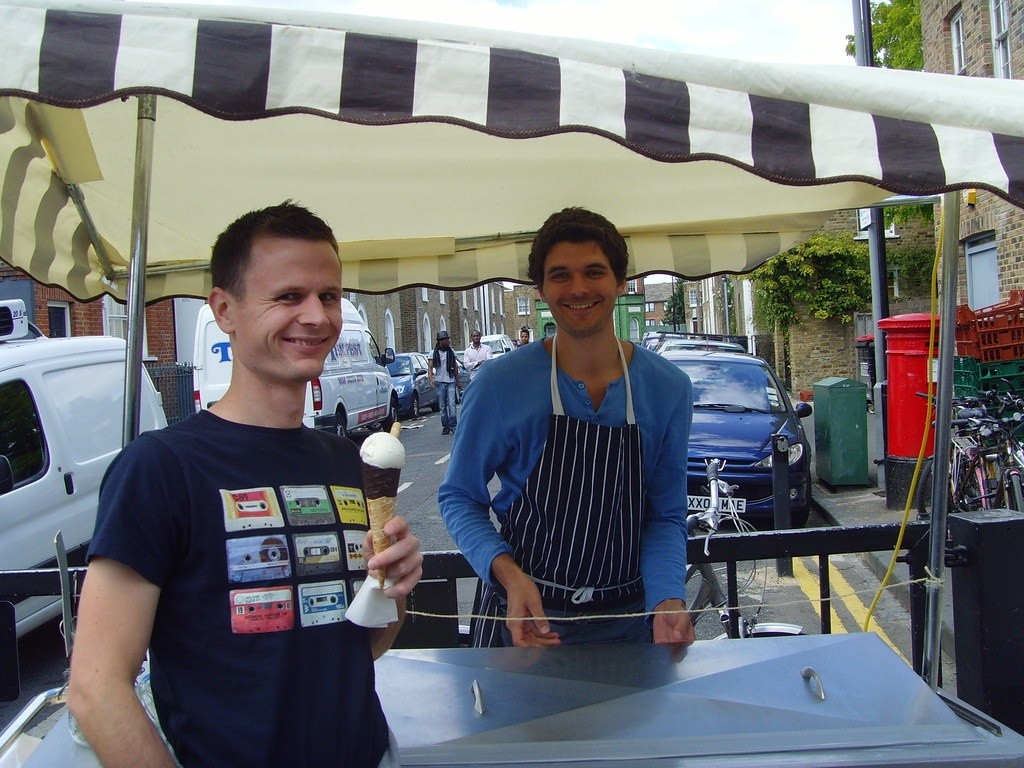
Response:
[427,331,461,435]
[517,329,531,348]
[436,206,695,648]
[66,199,425,768]
[463,330,492,382]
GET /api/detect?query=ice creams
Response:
[360,421,406,590]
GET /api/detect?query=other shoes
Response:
[442,427,451,435]
[449,426,456,433]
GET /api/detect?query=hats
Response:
[436,331,450,340]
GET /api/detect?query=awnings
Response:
[0,0,1024,689]
